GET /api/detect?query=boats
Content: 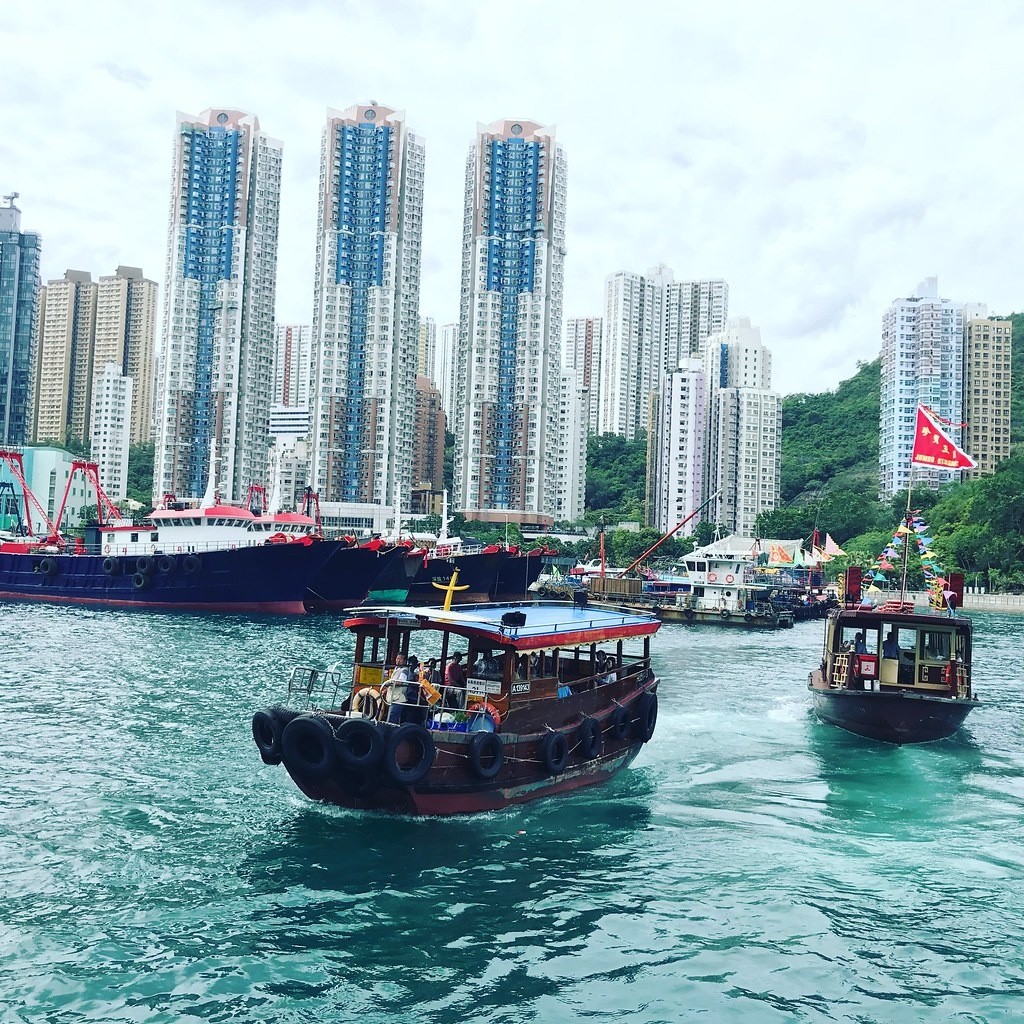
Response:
[809,589,983,744]
[0,445,555,621]
[244,568,664,817]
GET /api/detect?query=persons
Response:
[883,633,900,660]
[844,632,867,654]
[381,651,617,725]
[929,649,962,662]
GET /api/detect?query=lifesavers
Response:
[337,720,385,766]
[352,688,385,721]
[385,723,437,783]
[541,730,570,775]
[706,573,716,581]
[249,706,279,753]
[464,701,500,733]
[580,719,603,762]
[536,603,779,623]
[104,544,111,554]
[614,706,633,741]
[470,732,506,780]
[280,714,335,774]
[785,600,836,620]
[39,555,203,590]
[636,691,659,742]
[150,544,155,552]
[725,575,734,583]
